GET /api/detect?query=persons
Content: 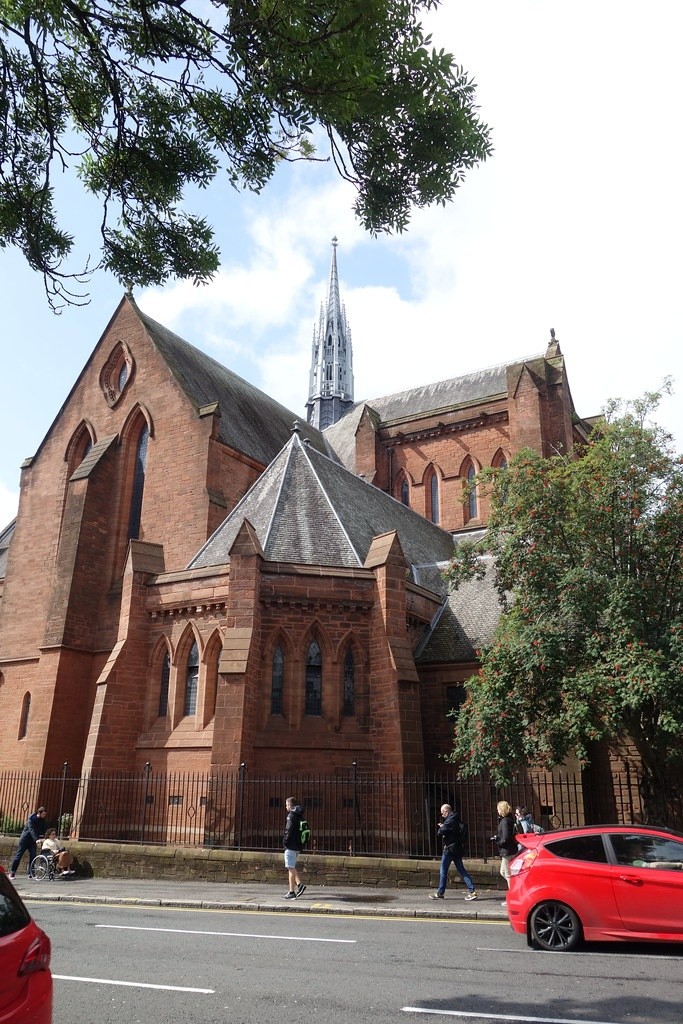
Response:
[428,804,478,900]
[282,797,308,901]
[514,805,535,851]
[495,801,524,906]
[9,807,49,880]
[41,828,76,877]
[625,837,683,871]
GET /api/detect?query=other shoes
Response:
[428,892,444,900]
[28,874,38,879]
[282,893,297,901]
[501,901,507,906]
[465,891,477,901]
[296,884,306,897]
[9,873,16,880]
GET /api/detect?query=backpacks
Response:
[458,821,468,844]
[530,823,545,833]
[513,822,524,846]
[290,811,311,845]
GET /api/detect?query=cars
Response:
[506,823,683,953]
[0,866,56,1024]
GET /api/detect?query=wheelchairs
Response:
[30,840,75,881]
